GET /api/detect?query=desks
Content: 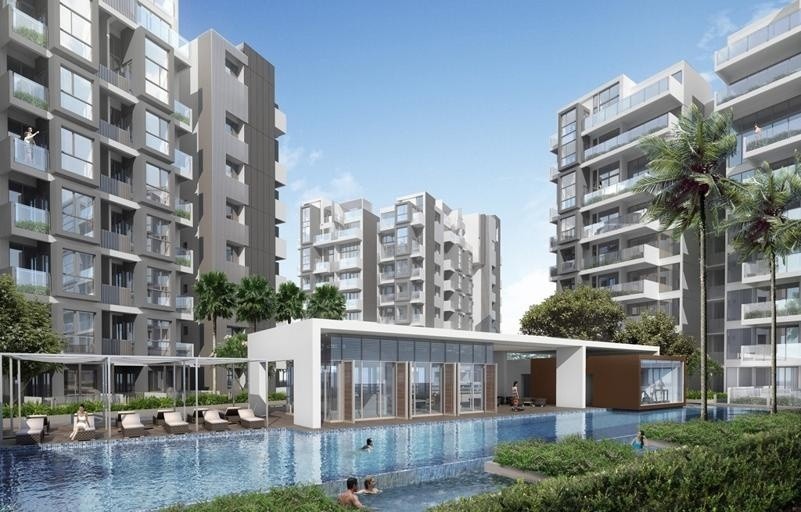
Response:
[497,396,547,408]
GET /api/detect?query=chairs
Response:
[16,407,265,445]
